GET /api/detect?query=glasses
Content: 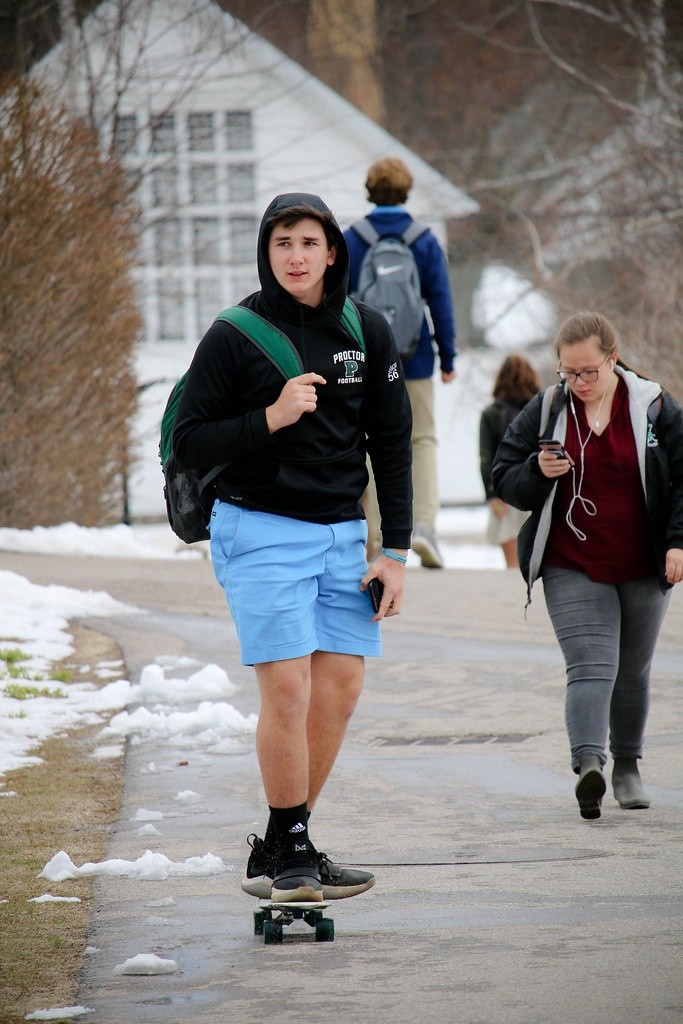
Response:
[557,354,611,383]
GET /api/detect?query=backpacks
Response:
[157,295,368,545]
[353,217,430,358]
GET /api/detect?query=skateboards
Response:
[252,901,335,946]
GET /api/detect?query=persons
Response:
[479,355,542,568]
[342,157,456,568]
[172,192,414,903]
[493,312,683,820]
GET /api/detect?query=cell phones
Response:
[368,577,384,613]
[539,440,567,460]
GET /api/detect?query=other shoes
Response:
[411,522,443,569]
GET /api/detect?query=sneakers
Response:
[270,838,323,901]
[241,833,376,899]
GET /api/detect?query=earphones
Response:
[610,358,613,371]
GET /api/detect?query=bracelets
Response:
[382,549,407,563]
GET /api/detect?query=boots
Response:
[574,754,607,819]
[612,756,650,808]
